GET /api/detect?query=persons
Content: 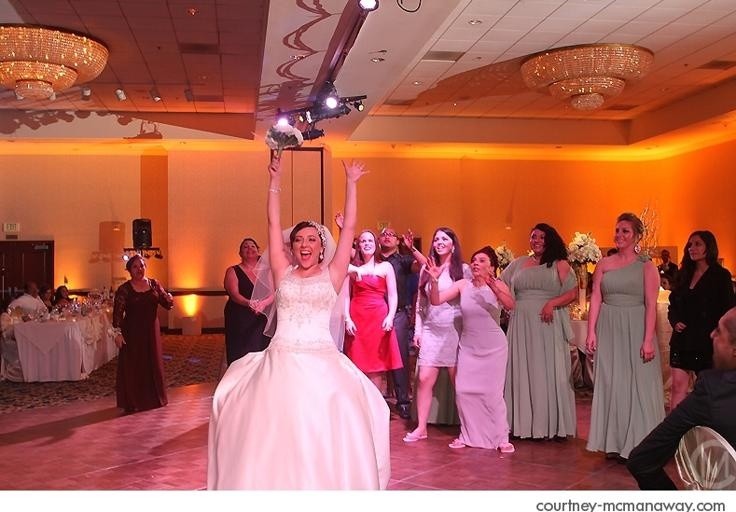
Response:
[496,222,580,442]
[216,237,272,366]
[585,213,668,464]
[7,280,72,316]
[340,228,404,419]
[421,245,516,454]
[334,212,428,419]
[606,228,732,415]
[403,226,474,443]
[109,253,174,412]
[206,153,392,490]
[625,307,736,491]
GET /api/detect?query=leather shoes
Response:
[399,405,410,418]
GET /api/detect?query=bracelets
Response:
[430,279,439,283]
[410,245,417,252]
[269,187,283,193]
[343,319,353,323]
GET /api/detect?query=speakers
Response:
[133,217,152,247]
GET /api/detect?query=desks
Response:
[12,301,126,383]
[566,302,669,357]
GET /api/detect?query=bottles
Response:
[109,286,113,299]
[103,287,107,296]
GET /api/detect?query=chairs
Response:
[673,426,736,490]
[0,312,25,382]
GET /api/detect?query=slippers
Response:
[500,443,514,453]
[448,439,464,449]
[402,433,426,442]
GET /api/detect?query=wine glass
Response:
[15,293,103,324]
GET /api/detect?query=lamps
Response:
[298,113,306,123]
[325,87,341,111]
[0,22,111,104]
[305,107,319,124]
[83,87,92,100]
[149,88,161,100]
[116,89,127,103]
[518,41,655,113]
[356,0,380,12]
[352,99,365,112]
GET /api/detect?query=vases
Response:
[576,261,589,306]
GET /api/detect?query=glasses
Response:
[382,232,396,237]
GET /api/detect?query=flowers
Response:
[563,230,603,265]
[263,122,304,161]
[493,245,515,271]
